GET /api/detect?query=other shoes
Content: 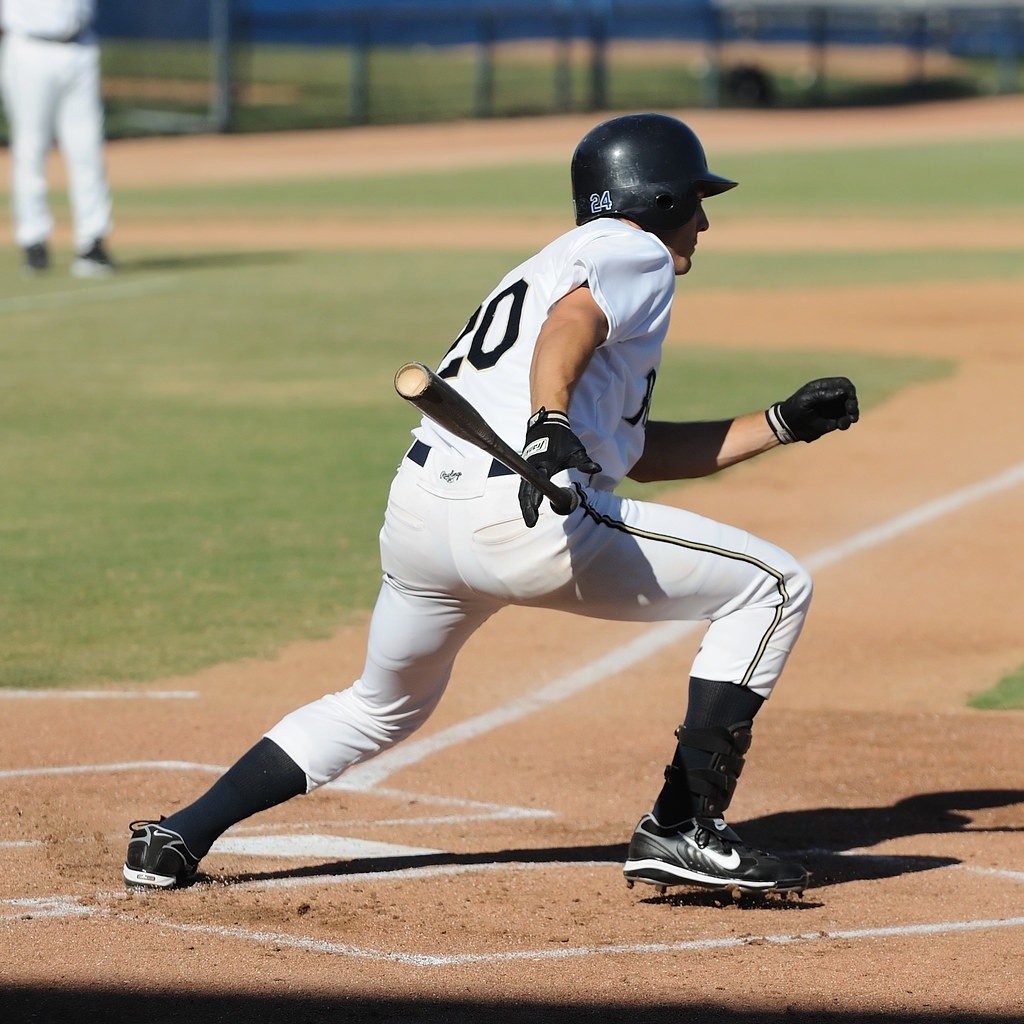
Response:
[27,244,47,271]
[82,247,113,266]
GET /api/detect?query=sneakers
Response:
[623,812,809,899]
[123,816,200,890]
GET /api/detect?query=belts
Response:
[407,440,516,478]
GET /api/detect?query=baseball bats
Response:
[391,357,586,519]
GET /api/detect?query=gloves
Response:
[766,377,859,445]
[518,406,604,528]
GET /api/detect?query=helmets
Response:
[571,113,740,226]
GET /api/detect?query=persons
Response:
[1,0,118,284]
[118,111,863,899]
